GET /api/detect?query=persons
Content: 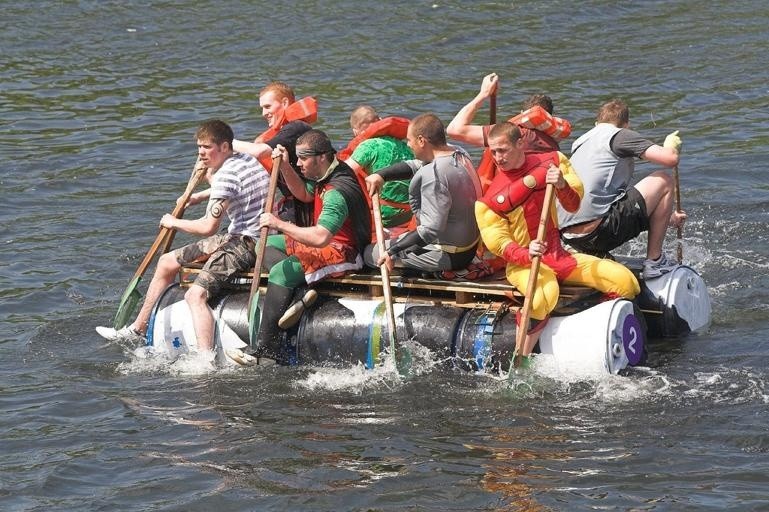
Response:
[363,114,483,273]
[446,72,571,197]
[474,121,641,357]
[95,120,279,373]
[196,82,313,234]
[555,97,688,279]
[226,128,371,368]
[342,105,418,228]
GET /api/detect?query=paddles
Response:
[372,192,413,381]
[113,168,208,330]
[507,182,555,384]
[248,155,282,347]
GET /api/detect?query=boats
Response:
[145,255,711,380]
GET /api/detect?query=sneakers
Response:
[95,326,148,359]
[441,265,485,280]
[507,356,536,383]
[226,345,276,366]
[278,289,317,329]
[471,256,493,275]
[642,252,679,279]
[565,244,615,262]
[172,353,215,373]
